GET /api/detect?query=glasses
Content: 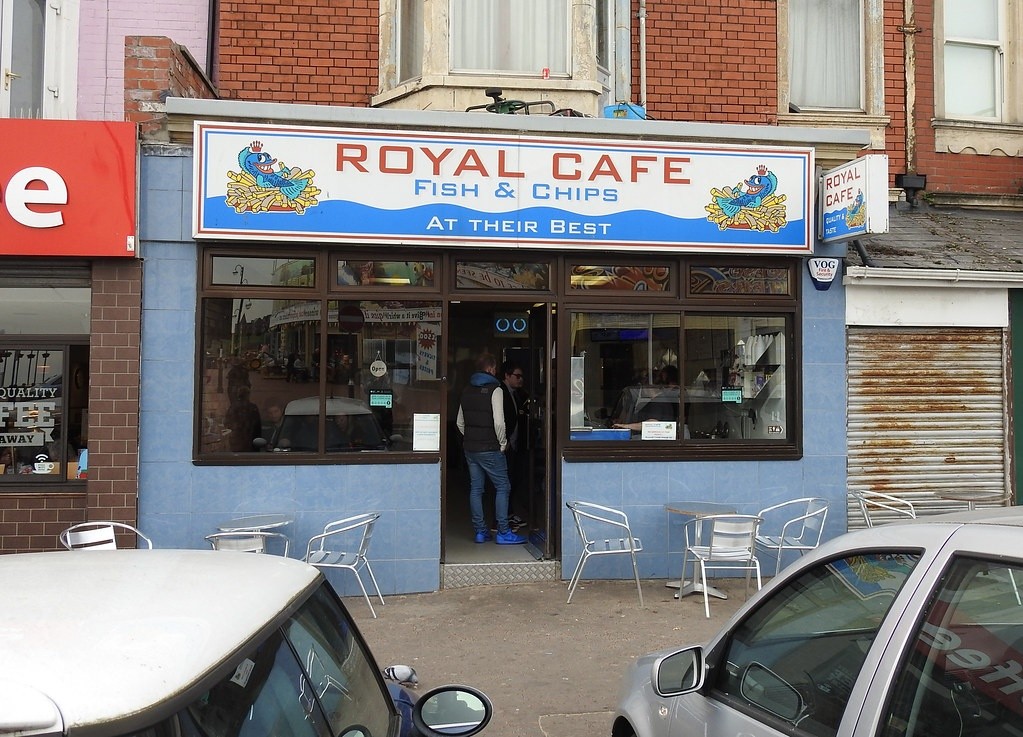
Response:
[511,372,524,378]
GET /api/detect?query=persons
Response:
[362,361,401,442]
[262,398,296,453]
[456,354,528,544]
[611,367,675,436]
[333,414,364,452]
[489,363,528,533]
[660,364,691,440]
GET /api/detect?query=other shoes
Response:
[491,520,519,532]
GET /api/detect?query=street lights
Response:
[231,262,252,362]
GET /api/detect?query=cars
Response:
[0,546,494,737]
[612,384,743,440]
[610,505,1023,737]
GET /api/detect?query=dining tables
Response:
[937,485,1012,511]
[216,513,297,533]
[666,500,739,601]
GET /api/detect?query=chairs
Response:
[740,495,830,602]
[301,511,385,620]
[675,513,763,617]
[850,488,917,526]
[56,519,153,555]
[203,530,291,560]
[560,497,648,613]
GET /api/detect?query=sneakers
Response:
[506,515,527,526]
[474,528,493,542]
[496,529,527,544]
